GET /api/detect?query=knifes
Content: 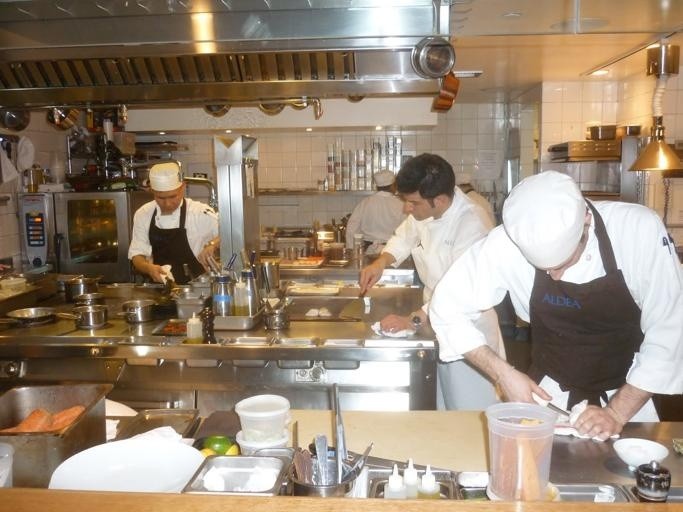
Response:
[309,441,451,471]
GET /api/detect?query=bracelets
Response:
[207,240,215,246]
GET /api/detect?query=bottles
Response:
[184,311,202,342]
[67,104,136,189]
[317,135,403,190]
[262,224,364,266]
[27,163,50,191]
[418,463,442,500]
[383,463,405,499]
[636,460,672,501]
[202,306,216,343]
[403,458,420,500]
[211,268,259,316]
[0,442,15,487]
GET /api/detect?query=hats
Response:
[501,170,587,269]
[148,162,183,192]
[372,169,396,188]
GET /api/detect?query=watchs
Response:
[410,311,422,330]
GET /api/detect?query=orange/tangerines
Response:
[203,434,232,454]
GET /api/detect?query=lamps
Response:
[626,43,682,173]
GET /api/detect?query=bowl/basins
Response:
[4,107,30,132]
[65,278,155,330]
[611,436,670,472]
[431,71,460,112]
[45,105,80,130]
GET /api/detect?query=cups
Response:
[485,401,558,501]
[235,392,291,441]
[258,262,279,289]
[264,310,289,330]
[233,426,290,456]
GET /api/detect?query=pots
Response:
[411,1,456,79]
[0,306,80,328]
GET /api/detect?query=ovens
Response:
[17,192,154,282]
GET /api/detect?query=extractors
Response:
[0,2,451,107]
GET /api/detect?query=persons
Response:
[358,152,507,410]
[344,171,410,270]
[453,172,496,228]
[427,168,681,441]
[122,161,220,282]
